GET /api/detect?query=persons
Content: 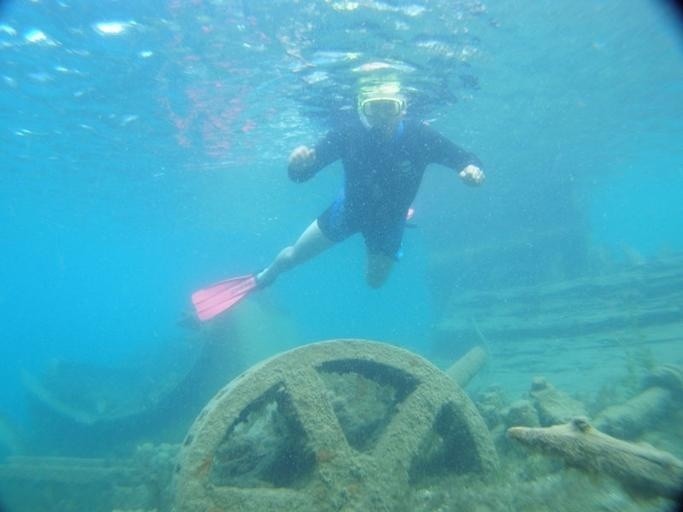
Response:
[189,74,486,323]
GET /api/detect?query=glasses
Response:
[362,98,404,116]
